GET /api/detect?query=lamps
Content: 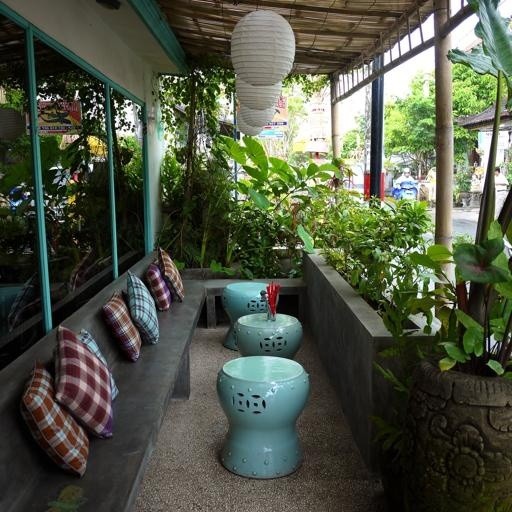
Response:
[230,0,296,137]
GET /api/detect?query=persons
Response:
[390,166,414,192]
[494,167,510,220]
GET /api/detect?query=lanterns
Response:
[237,103,279,128]
[231,10,297,87]
[233,77,283,108]
[234,117,265,137]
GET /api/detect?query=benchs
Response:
[1,246,143,368]
[0,248,207,511]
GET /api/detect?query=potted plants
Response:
[399,0,512,512]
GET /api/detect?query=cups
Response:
[266,303,277,322]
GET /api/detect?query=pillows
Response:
[19,359,90,477]
[50,329,119,401]
[145,259,172,311]
[8,247,114,330]
[158,246,186,302]
[52,324,114,440]
[101,288,142,361]
[124,269,159,345]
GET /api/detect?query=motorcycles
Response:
[392,181,418,200]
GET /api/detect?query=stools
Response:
[221,282,279,351]
[215,354,310,479]
[232,313,303,359]
